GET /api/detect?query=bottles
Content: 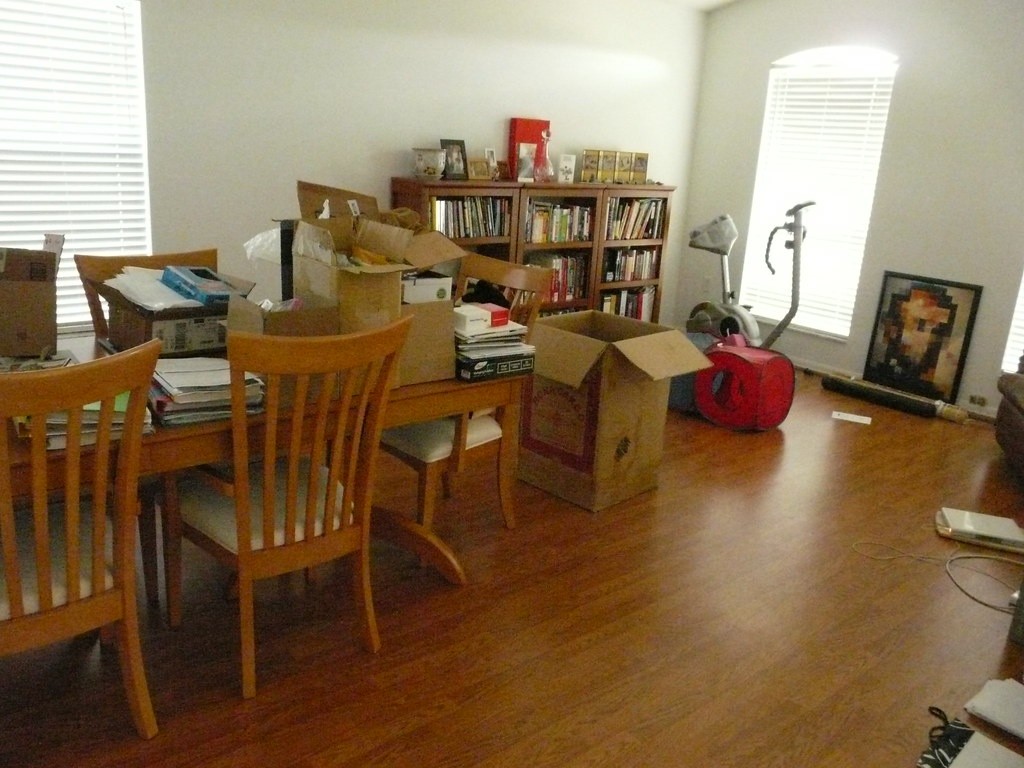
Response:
[534,130,554,182]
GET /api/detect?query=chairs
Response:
[162,315,411,696]
[380,247,553,570]
[0,338,160,739]
[73,248,217,336]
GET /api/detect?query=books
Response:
[963,679,1024,739]
[0,355,264,450]
[432,197,664,350]
[936,506,1024,555]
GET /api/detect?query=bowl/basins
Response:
[412,147,447,179]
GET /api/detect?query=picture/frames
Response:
[441,139,469,180]
[466,160,491,179]
[862,270,984,404]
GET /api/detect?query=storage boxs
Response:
[80,265,256,351]
[1,231,65,354]
[243,180,469,399]
[521,308,713,512]
[455,345,536,381]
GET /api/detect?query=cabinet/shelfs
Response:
[389,175,675,321]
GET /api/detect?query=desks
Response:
[0,331,528,529]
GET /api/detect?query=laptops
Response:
[940,507,1024,548]
